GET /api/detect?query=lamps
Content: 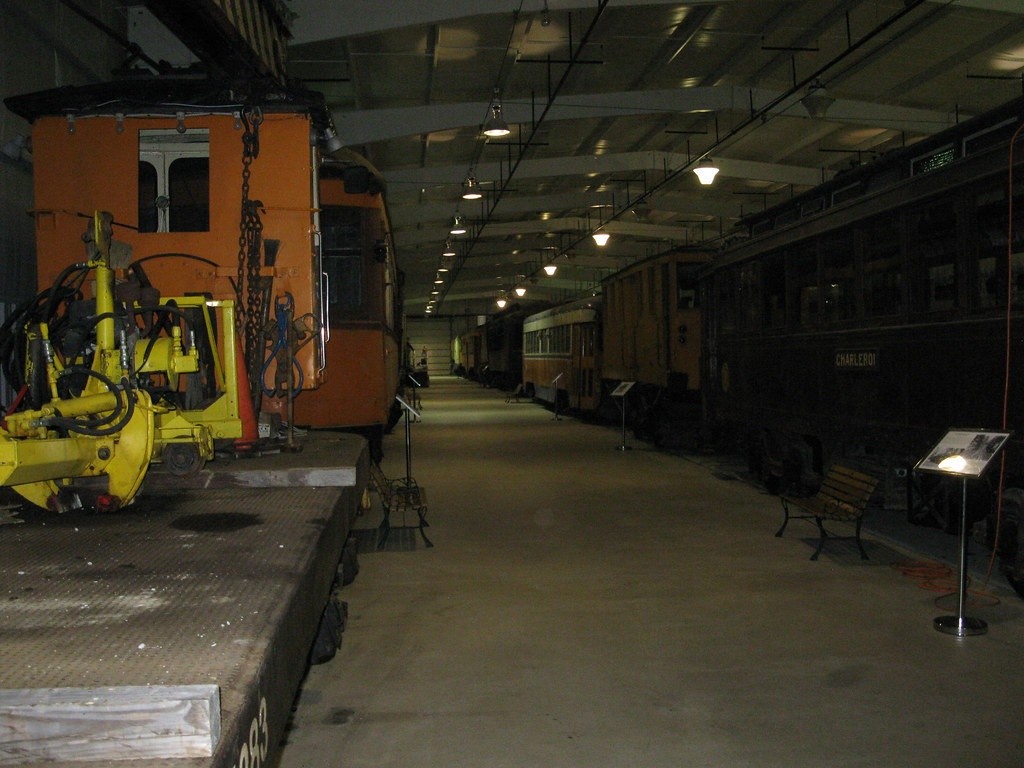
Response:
[515,284,526,297]
[591,228,610,246]
[450,217,466,234]
[544,262,556,275]
[482,106,509,140]
[632,201,651,221]
[692,158,719,185]
[497,298,506,308]
[434,274,444,284]
[461,177,482,200]
[437,263,448,272]
[442,241,456,257]
[801,84,835,121]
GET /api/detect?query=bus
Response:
[450,94,1024,546]
[261,161,414,429]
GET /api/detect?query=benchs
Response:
[775,464,879,560]
[370,461,433,549]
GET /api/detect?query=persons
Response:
[454,363,465,376]
[406,337,413,351]
[449,358,455,375]
